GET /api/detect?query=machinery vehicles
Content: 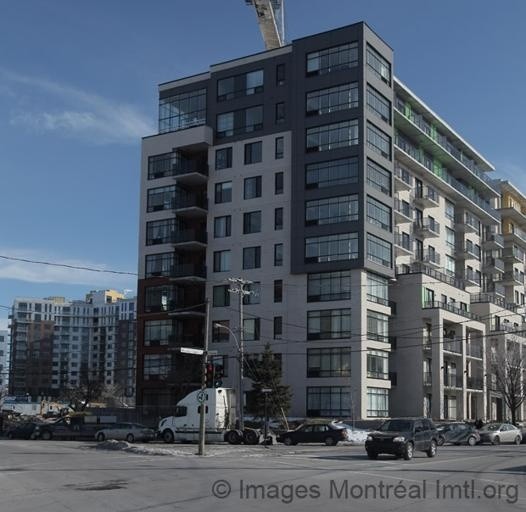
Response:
[37,399,70,421]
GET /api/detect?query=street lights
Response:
[214,323,287,430]
[167,311,210,456]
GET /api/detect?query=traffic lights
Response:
[205,362,223,388]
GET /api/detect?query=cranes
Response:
[243,0,284,51]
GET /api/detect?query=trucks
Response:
[157,387,278,445]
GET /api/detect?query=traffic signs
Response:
[180,347,217,357]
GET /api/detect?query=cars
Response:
[365,419,526,460]
[276,423,348,446]
[5,413,157,444]
